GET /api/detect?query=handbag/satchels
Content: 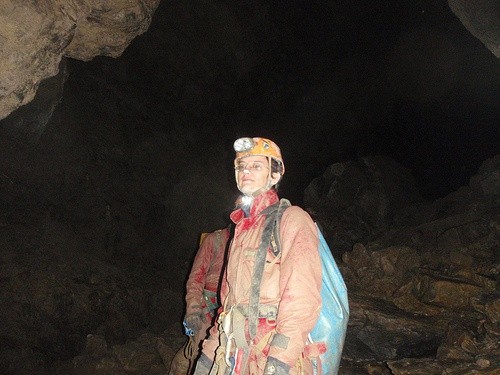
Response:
[303,222,350,375]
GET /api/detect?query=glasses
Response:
[233,162,269,172]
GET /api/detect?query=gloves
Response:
[193,352,214,375]
[183,307,208,331]
[263,356,291,375]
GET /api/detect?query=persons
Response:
[184,222,231,355]
[194,137,349,375]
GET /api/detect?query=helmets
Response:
[233,136,286,176]
